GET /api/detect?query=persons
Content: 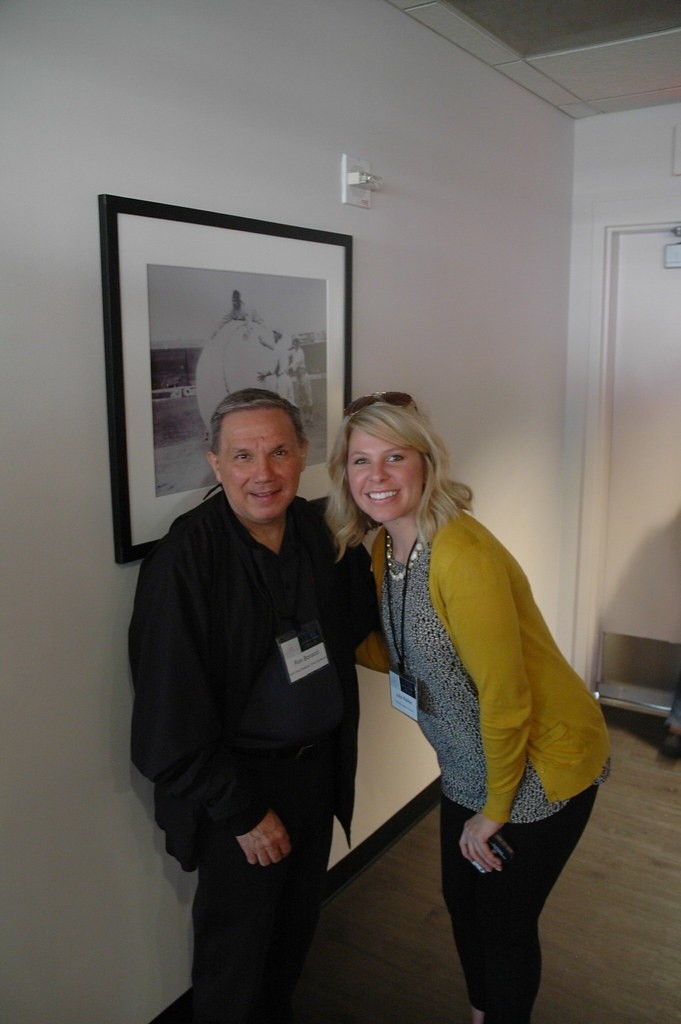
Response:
[258,327,294,403]
[286,339,313,408]
[325,392,613,1024]
[210,289,269,340]
[127,390,378,1024]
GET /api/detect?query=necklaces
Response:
[386,535,423,581]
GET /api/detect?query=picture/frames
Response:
[98,193,353,566]
